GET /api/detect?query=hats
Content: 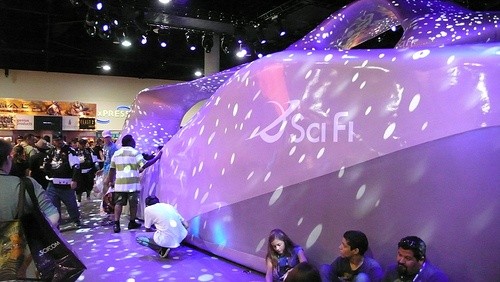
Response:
[53,131,63,140]
[17,135,23,141]
[102,130,112,138]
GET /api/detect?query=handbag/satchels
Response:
[21,178,87,282]
[0,178,26,282]
[100,186,115,217]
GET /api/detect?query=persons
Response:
[282,261,323,282]
[0,139,60,279]
[384,236,450,282]
[380,266,414,282]
[328,258,355,282]
[10,129,162,233]
[136,195,189,257]
[265,229,307,282]
[318,230,384,282]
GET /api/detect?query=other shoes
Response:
[159,248,171,259]
[74,221,81,227]
[86,197,90,203]
[101,217,115,225]
[76,201,82,207]
[128,222,141,229]
[113,225,121,233]
